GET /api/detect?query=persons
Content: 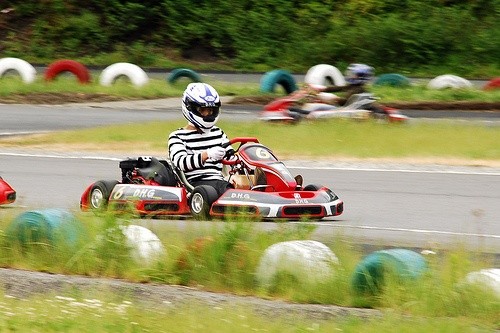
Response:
[168,82,238,197]
[308,63,373,106]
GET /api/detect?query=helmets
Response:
[182,83,221,129]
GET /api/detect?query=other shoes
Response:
[294,175,303,191]
[253,167,266,192]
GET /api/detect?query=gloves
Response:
[206,146,226,160]
